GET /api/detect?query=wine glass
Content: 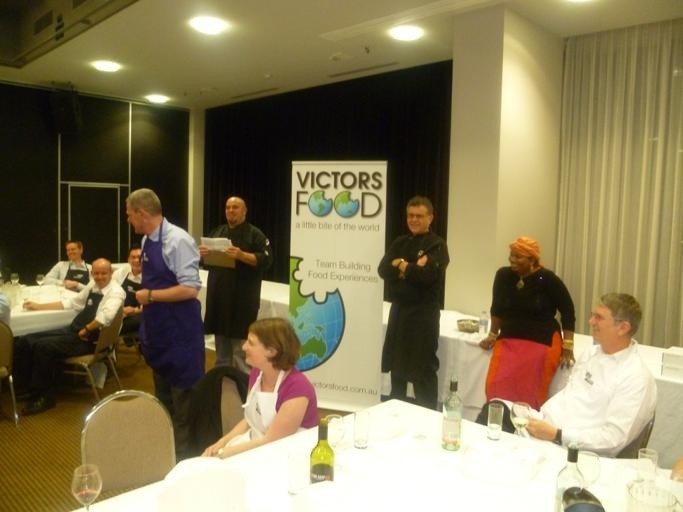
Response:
[0,270,67,307]
[71,463,102,511]
[509,401,531,456]
[327,413,345,469]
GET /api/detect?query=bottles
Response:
[554,436,583,499]
[442,376,464,452]
[479,309,489,341]
[310,418,335,483]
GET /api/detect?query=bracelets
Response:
[147,289,153,304]
[217,448,224,460]
[396,258,404,269]
[84,324,91,333]
[488,331,498,340]
[562,339,574,350]
[551,427,562,445]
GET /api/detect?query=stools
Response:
[119,331,142,362]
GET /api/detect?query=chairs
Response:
[619,409,657,458]
[180,366,251,457]
[0,320,19,425]
[63,303,123,403]
[79,386,175,495]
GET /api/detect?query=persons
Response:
[472,292,660,465]
[110,245,145,336]
[40,238,93,293]
[124,186,208,465]
[198,316,322,462]
[9,256,125,414]
[477,236,579,408]
[377,194,451,411]
[198,194,275,378]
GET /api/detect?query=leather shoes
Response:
[16,389,56,416]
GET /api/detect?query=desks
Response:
[382,300,681,468]
[106,262,288,361]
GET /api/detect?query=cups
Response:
[351,408,369,449]
[628,481,683,512]
[486,401,505,441]
[638,447,659,485]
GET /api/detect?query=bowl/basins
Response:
[455,320,480,332]
